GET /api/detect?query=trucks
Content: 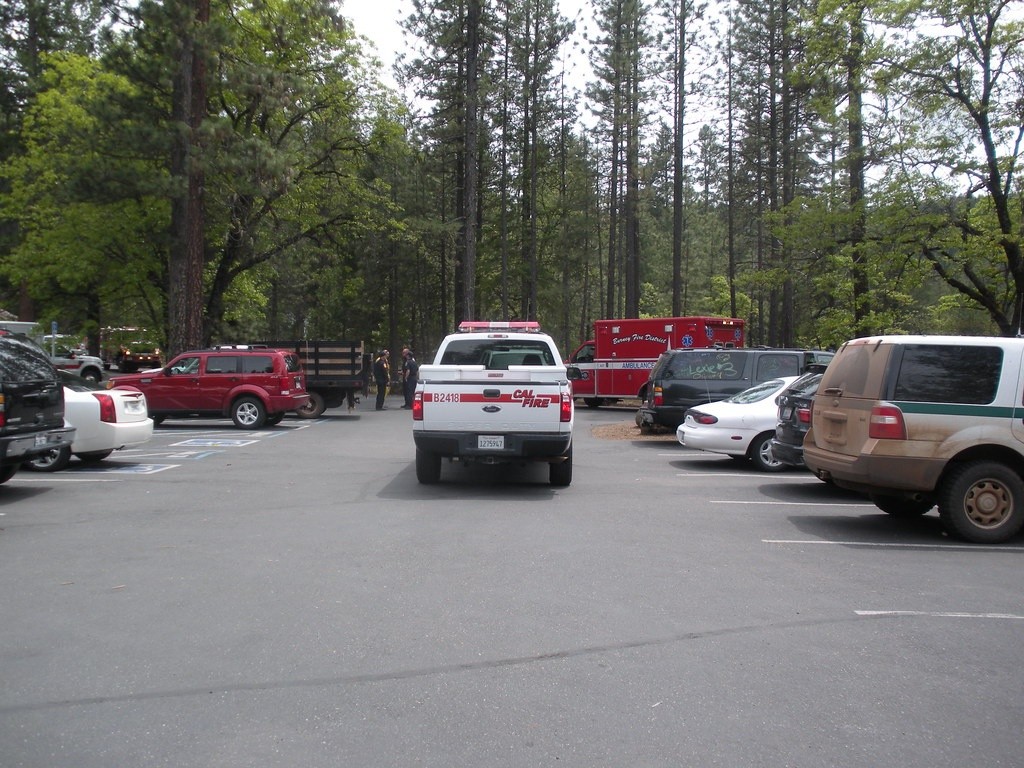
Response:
[0,321,104,386]
[220,336,370,420]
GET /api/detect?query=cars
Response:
[677,376,801,473]
[25,365,154,472]
[42,331,88,356]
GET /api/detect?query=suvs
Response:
[770,362,863,501]
[0,328,76,482]
[109,347,311,429]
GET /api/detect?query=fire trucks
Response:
[564,317,744,406]
[85,324,161,371]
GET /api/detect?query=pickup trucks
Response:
[638,348,839,432]
[411,320,574,490]
[803,335,1024,547]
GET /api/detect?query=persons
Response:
[401,349,418,410]
[374,350,390,412]
